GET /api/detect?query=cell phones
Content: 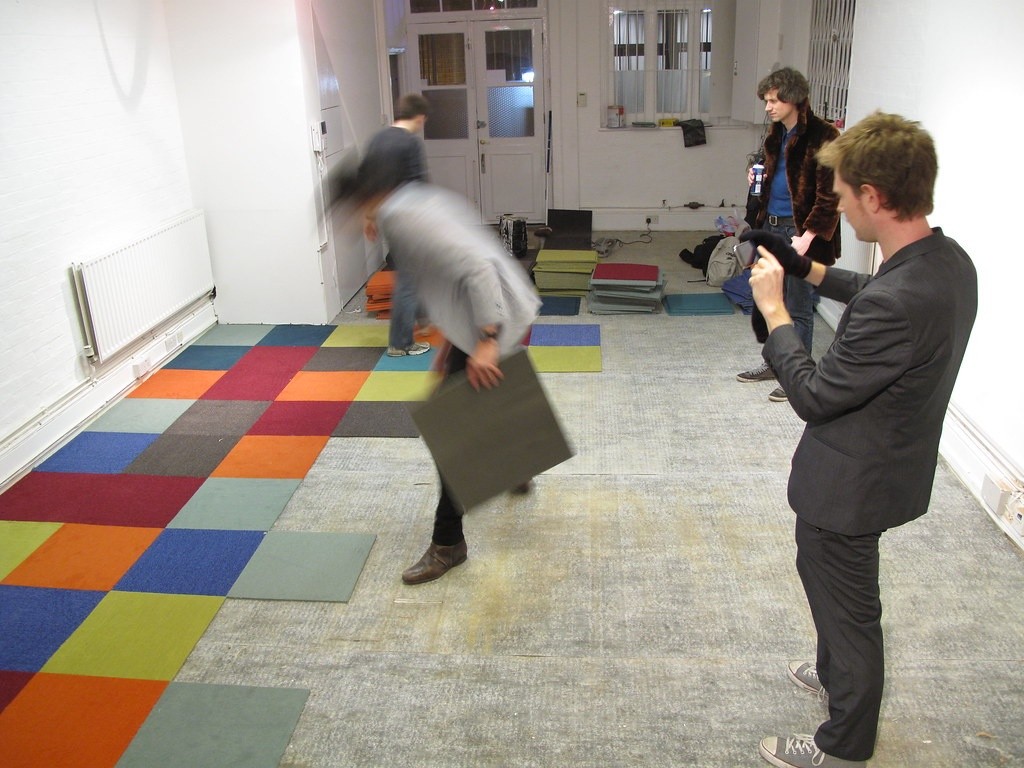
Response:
[734,239,761,270]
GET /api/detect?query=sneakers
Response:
[387,341,430,357]
[416,320,436,335]
[788,660,830,702]
[758,733,867,767]
[736,361,775,382]
[769,386,788,401]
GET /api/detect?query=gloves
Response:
[739,229,812,279]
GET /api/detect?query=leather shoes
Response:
[402,540,467,584]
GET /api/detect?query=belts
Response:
[766,214,793,227]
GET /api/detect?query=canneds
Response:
[608,105,624,128]
[750,164,765,196]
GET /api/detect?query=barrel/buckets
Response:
[606,105,624,128]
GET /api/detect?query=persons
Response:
[322,95,544,587]
[740,110,979,768]
[735,66,842,402]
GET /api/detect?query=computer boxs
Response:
[499,217,527,258]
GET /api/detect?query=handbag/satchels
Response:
[496,214,529,260]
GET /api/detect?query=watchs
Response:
[479,329,495,343]
